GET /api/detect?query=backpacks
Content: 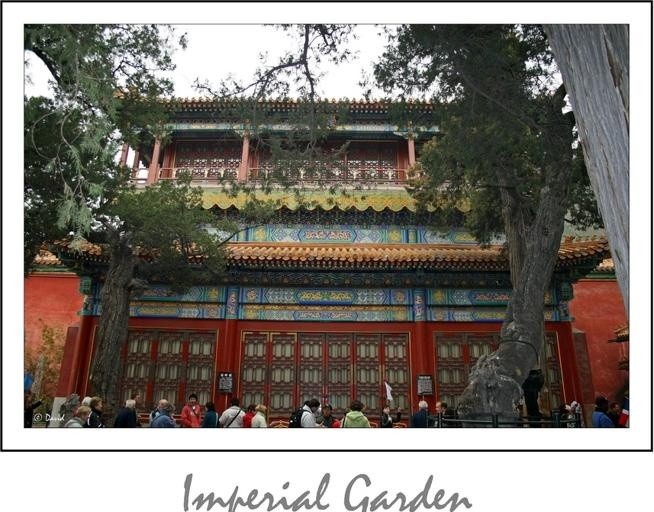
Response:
[289,407,311,427]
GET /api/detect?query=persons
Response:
[24,394,629,428]
